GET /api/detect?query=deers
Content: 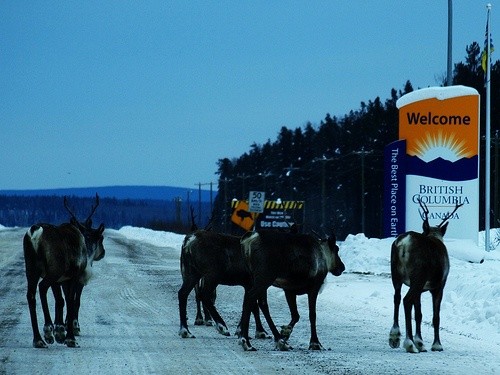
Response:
[178,195,345,352]
[387,199,464,354]
[23,192,106,349]
